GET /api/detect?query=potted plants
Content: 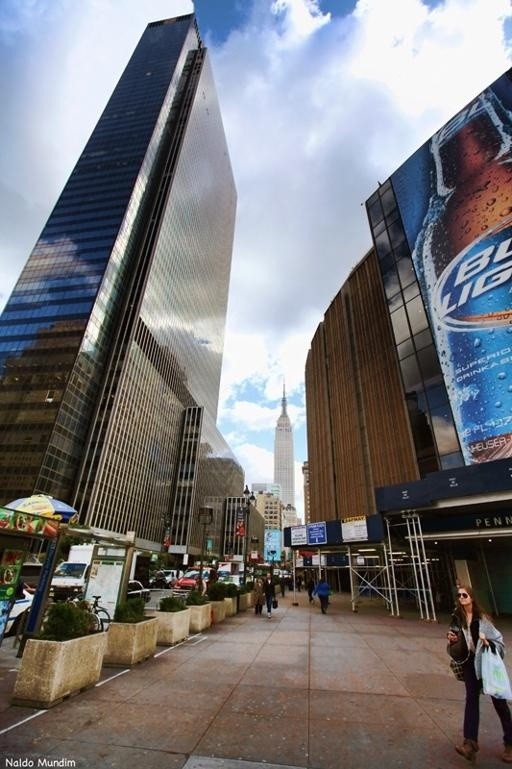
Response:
[11,582,254,707]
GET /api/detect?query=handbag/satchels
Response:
[481,646,512,701]
[447,639,469,682]
[272,596,278,608]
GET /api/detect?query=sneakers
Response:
[455,738,479,761]
[501,744,512,763]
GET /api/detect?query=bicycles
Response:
[67,587,112,636]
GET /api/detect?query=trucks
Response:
[48,544,138,602]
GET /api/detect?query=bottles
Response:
[422,95,512,463]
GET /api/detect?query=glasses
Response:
[457,593,470,598]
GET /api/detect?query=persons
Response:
[263,572,278,618]
[305,577,315,602]
[280,575,285,596]
[251,577,266,615]
[447,585,511,763]
[313,579,332,614]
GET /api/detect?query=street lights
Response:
[243,485,255,584]
[196,506,214,585]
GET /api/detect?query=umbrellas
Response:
[2,493,78,524]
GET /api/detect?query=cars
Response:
[274,568,291,578]
[148,565,218,590]
[127,580,152,603]
